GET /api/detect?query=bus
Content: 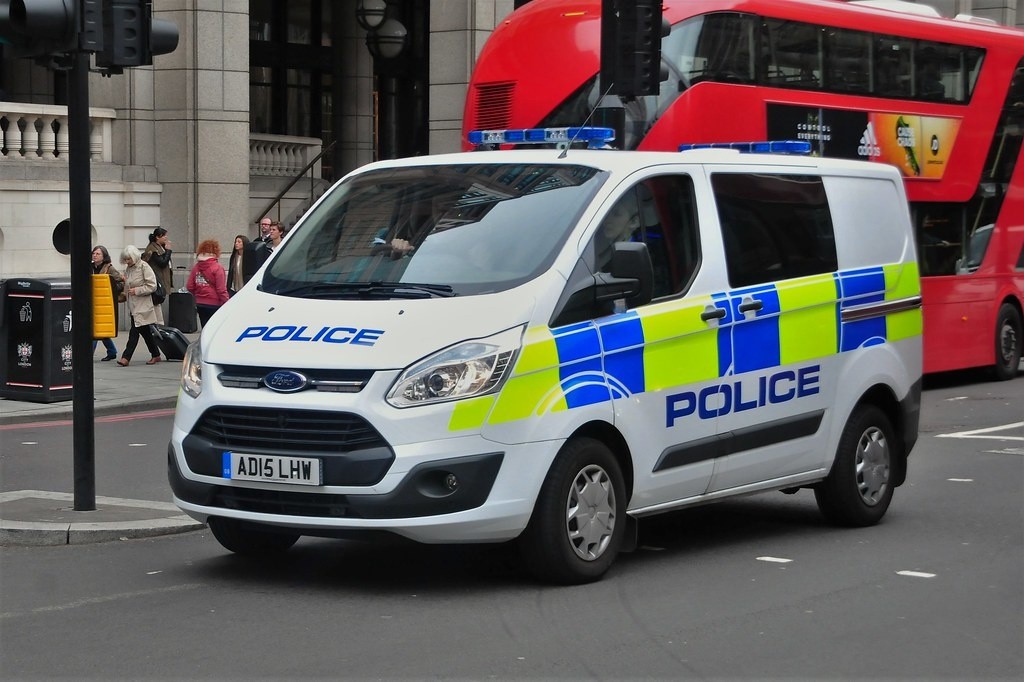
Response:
[461,0,1024,383]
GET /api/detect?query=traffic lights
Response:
[0,0,103,75]
[96,0,179,74]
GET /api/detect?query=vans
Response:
[168,79,925,585]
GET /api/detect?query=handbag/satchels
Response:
[228,288,236,298]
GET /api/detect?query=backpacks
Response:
[141,262,166,305]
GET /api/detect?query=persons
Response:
[915,47,943,104]
[91,245,124,361]
[389,188,464,264]
[875,47,899,99]
[186,240,229,329]
[267,221,285,247]
[254,217,273,244]
[226,236,258,298]
[141,228,174,326]
[596,193,641,274]
[117,245,161,366]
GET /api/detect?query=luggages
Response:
[149,323,190,360]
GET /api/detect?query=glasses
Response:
[261,223,270,227]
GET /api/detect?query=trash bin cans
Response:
[1,276,74,405]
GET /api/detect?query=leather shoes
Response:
[146,356,161,364]
[117,358,128,365]
[101,354,117,361]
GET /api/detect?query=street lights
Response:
[355,1,410,161]
